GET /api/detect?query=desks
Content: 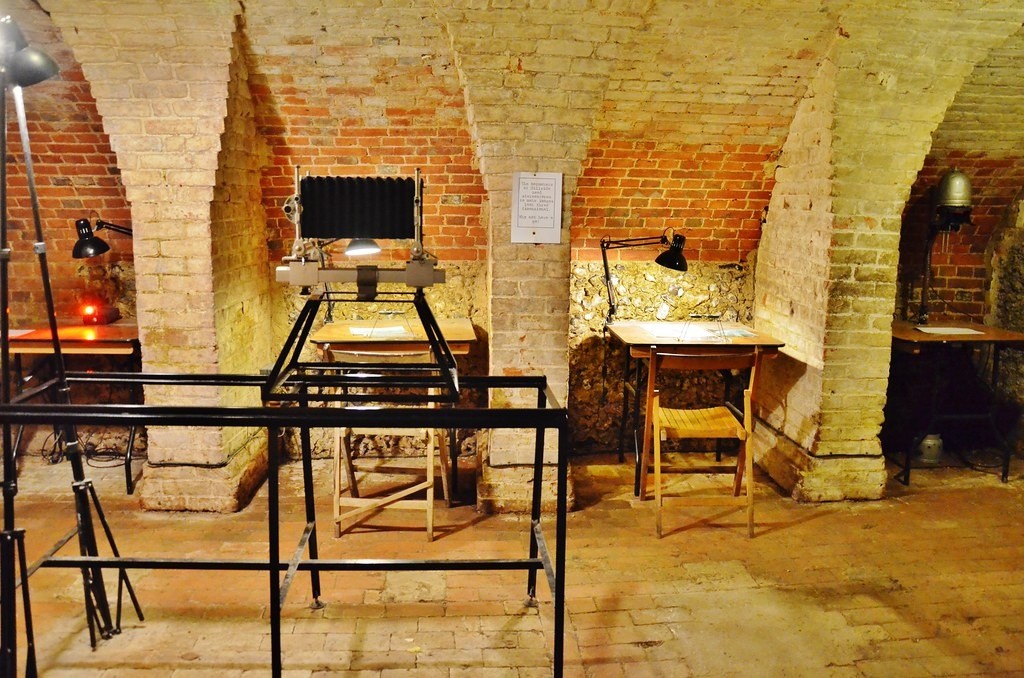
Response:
[310,317,478,495]
[7,326,144,495]
[891,320,1024,485]
[605,322,785,496]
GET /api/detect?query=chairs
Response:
[639,346,764,539]
[322,342,454,542]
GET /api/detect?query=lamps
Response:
[318,238,382,324]
[600,227,688,332]
[71,211,132,259]
[0,13,70,416]
